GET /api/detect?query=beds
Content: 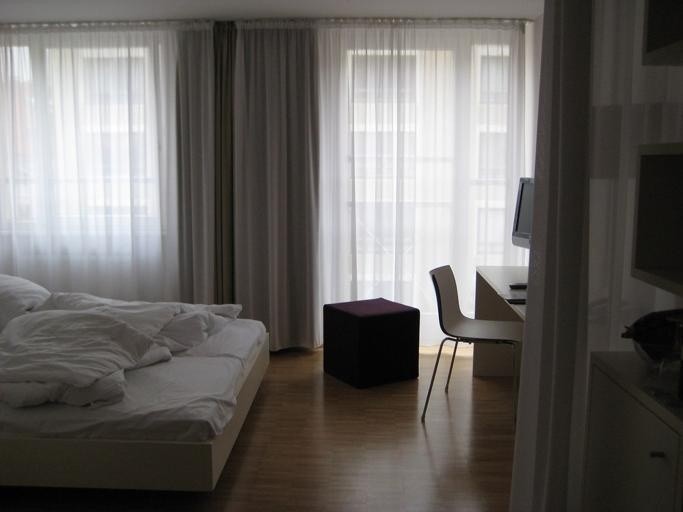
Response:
[0,274,271,491]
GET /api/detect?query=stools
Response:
[321,296,420,392]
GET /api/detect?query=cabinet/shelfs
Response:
[583,350,680,511]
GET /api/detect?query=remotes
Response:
[509,283,527,290]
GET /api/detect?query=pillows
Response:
[0,273,51,329]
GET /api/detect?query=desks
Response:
[471,269,525,378]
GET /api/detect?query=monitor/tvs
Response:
[512,177,534,248]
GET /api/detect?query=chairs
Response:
[421,265,524,432]
